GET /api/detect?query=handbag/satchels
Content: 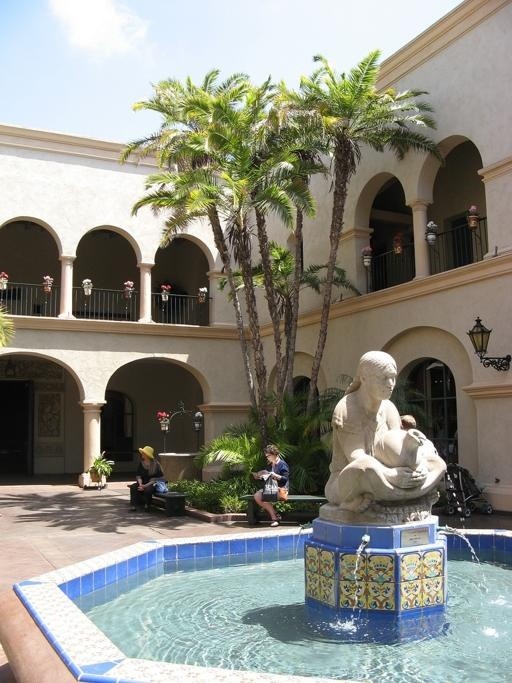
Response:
[262,474,280,502]
[154,479,169,493]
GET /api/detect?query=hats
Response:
[138,446,155,460]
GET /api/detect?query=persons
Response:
[323,349,448,510]
[130,445,164,514]
[252,444,290,526]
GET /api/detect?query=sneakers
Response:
[128,508,153,516]
[270,511,282,527]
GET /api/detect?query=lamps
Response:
[464,315,512,373]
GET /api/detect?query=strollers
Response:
[442,460,493,517]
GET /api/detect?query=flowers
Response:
[0,270,208,293]
[360,204,479,257]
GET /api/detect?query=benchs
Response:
[236,493,328,526]
[128,481,189,516]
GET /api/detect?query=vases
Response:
[362,256,373,267]
[83,284,207,303]
